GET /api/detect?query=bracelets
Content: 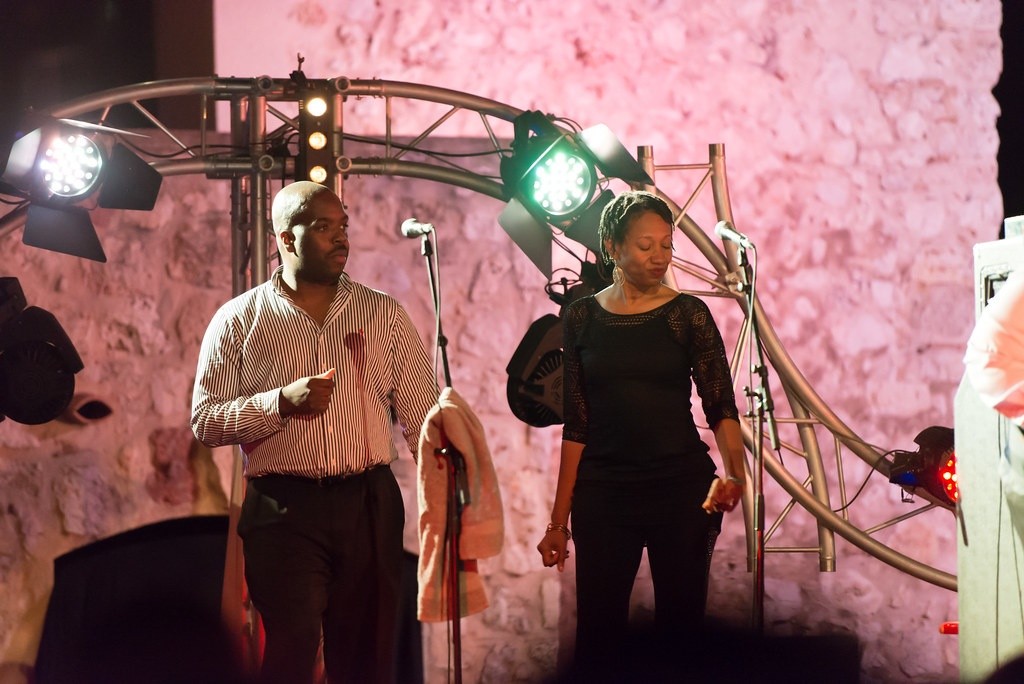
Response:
[724,474,746,485]
[544,523,572,541]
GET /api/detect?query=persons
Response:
[190,177,444,684]
[537,192,747,672]
[951,262,1023,682]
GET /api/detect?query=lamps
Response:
[889,425,961,507]
[0,99,163,263]
[497,110,655,282]
[297,78,337,189]
[0,276,84,425]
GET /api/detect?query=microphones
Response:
[714,220,755,249]
[401,218,434,239]
